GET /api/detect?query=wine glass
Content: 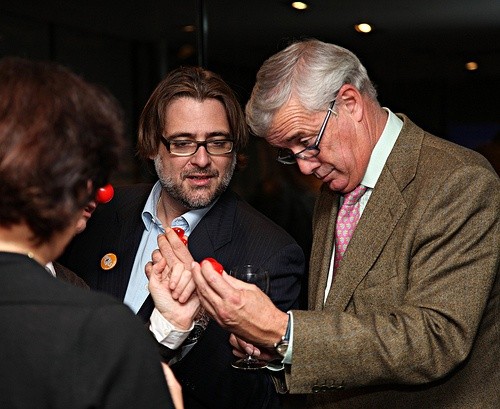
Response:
[229,265,269,370]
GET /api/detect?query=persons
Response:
[190,42,499,409]
[0,54,304,409]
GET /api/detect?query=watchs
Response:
[273,314,292,358]
[185,309,212,343]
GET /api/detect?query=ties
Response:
[334,182,368,284]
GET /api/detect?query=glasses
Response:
[159,135,234,156]
[274,91,338,164]
